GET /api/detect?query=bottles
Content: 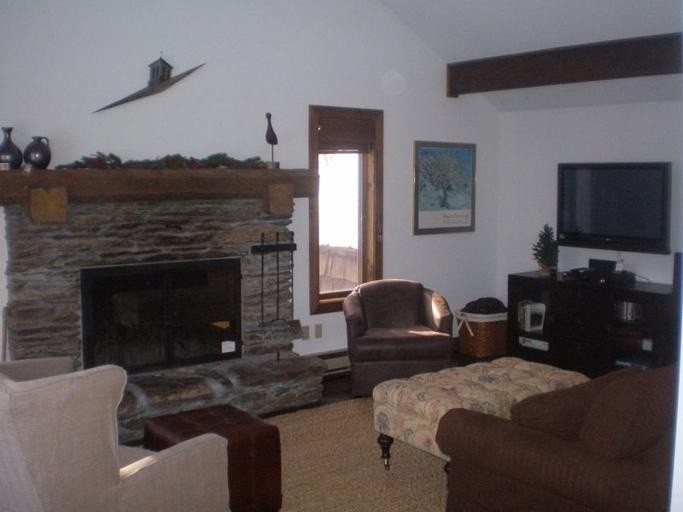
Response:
[0,126,23,168]
[22,136,51,168]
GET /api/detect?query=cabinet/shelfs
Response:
[501,268,671,368]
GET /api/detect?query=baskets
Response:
[456,310,508,359]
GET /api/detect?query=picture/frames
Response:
[410,137,477,234]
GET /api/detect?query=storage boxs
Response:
[457,306,510,364]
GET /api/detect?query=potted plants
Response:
[530,222,562,279]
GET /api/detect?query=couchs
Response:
[369,352,584,467]
[0,351,235,512]
[431,367,677,512]
[139,404,284,512]
[343,277,457,397]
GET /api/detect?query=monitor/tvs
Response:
[557,161,672,255]
